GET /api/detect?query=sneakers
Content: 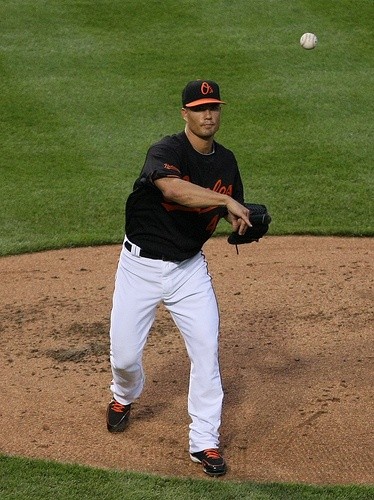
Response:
[104,397,131,434]
[189,448,227,476]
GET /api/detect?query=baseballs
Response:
[300,33,317,49]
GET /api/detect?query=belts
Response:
[124,241,188,262]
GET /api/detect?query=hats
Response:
[181,79,226,107]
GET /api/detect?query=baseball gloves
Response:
[228,203,271,244]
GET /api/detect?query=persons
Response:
[106,79,272,477]
